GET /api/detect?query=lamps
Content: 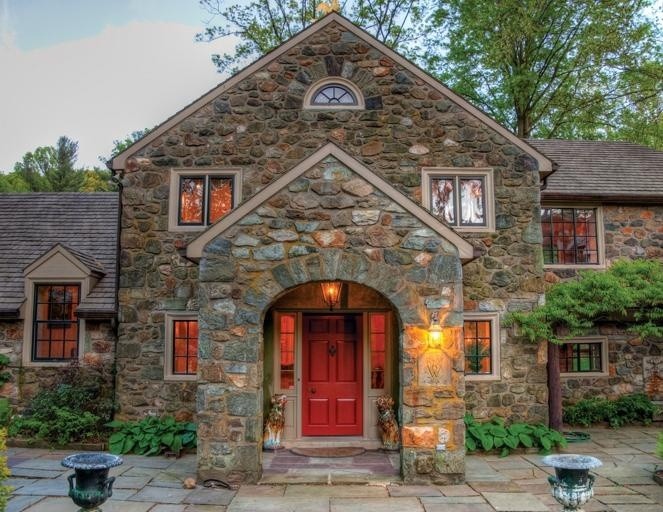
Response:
[320,281,343,308]
[426,313,444,350]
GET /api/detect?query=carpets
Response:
[289,446,369,458]
